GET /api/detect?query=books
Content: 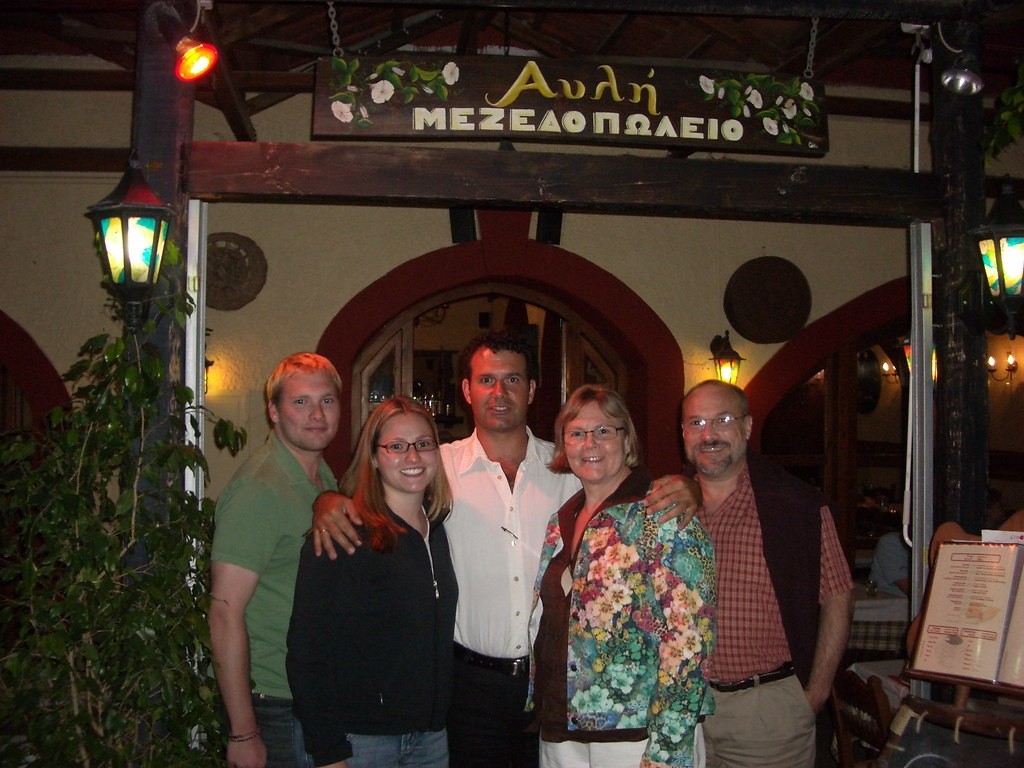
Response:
[913,545,1024,686]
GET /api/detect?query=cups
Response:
[866,580,878,596]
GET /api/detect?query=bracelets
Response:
[228,729,263,742]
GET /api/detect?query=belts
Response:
[710,668,795,693]
[454,645,529,679]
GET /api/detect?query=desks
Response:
[830,659,910,763]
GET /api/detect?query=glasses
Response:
[564,425,624,443]
[375,439,440,454]
[683,412,751,433]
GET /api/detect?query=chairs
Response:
[831,670,892,768]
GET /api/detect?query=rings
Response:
[319,529,328,534]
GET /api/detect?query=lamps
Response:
[708,329,746,386]
[414,304,450,328]
[883,362,900,384]
[82,162,177,325]
[987,352,1018,383]
[940,52,985,95]
[965,173,1024,340]
[144,0,219,84]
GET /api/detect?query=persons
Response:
[288,395,459,768]
[312,329,703,768]
[525,385,718,768]
[682,379,853,768]
[208,352,347,768]
